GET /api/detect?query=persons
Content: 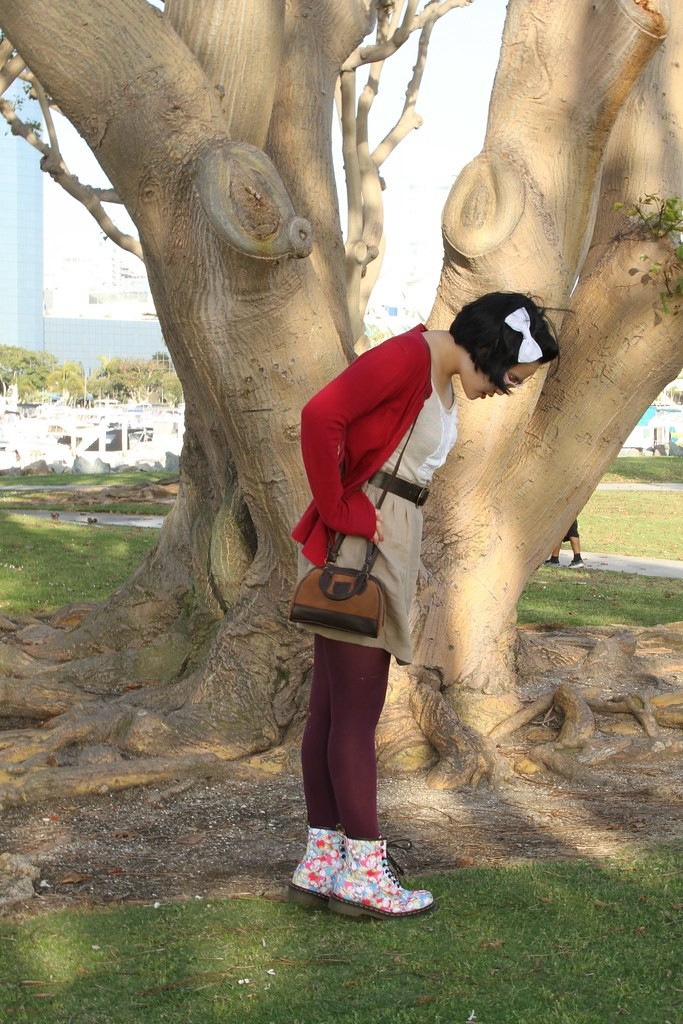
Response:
[545,518,584,568]
[288,291,577,920]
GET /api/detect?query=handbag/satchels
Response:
[288,533,386,638]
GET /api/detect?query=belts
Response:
[368,469,431,508]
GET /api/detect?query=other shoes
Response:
[546,559,559,566]
[569,560,584,568]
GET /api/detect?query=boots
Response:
[327,824,435,919]
[288,826,345,906]
